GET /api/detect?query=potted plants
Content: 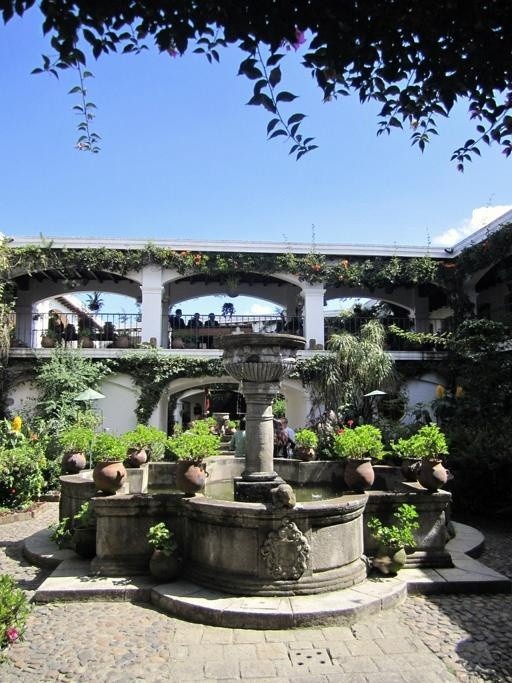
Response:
[295,428,319,461]
[140,521,183,585]
[359,501,421,578]
[332,424,387,493]
[51,412,245,495]
[389,421,454,491]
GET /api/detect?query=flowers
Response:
[6,627,21,644]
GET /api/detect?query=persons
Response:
[170,307,187,342]
[210,416,296,459]
[204,312,221,349]
[187,313,204,349]
